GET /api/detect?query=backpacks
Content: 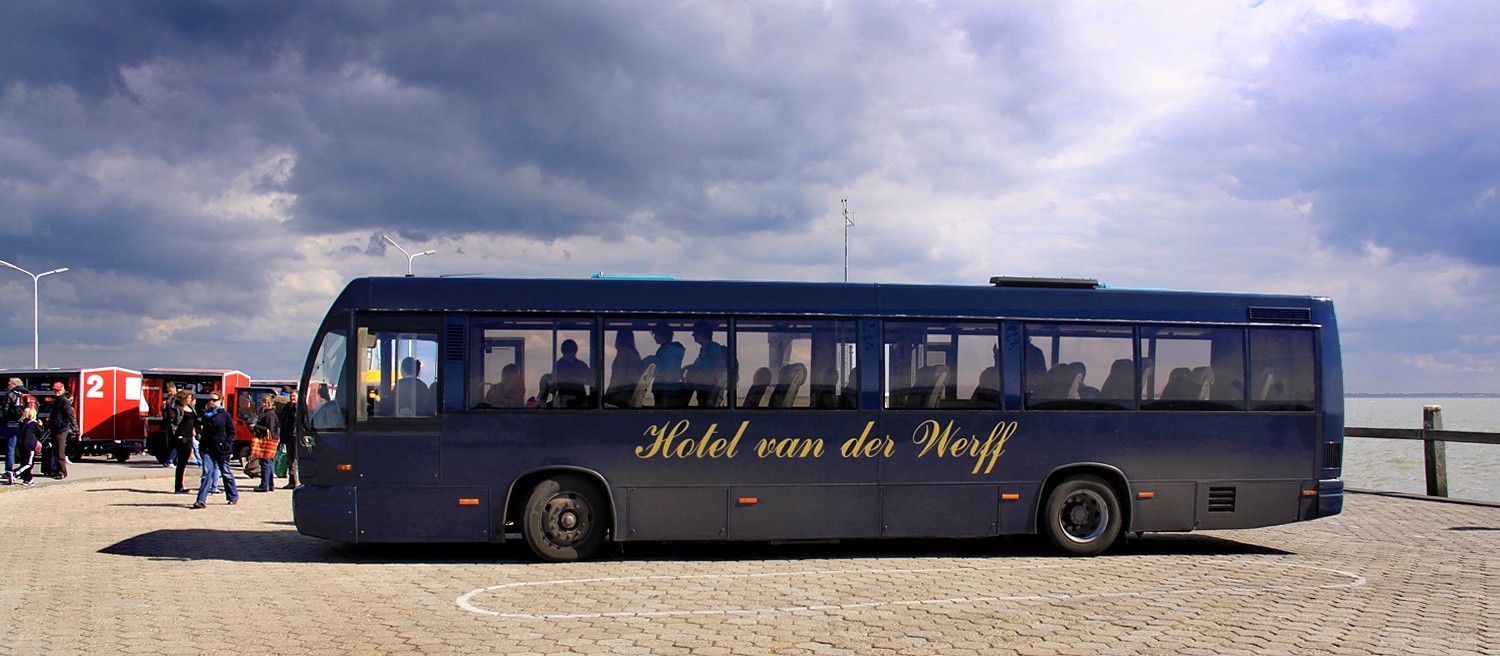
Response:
[11,390,37,421]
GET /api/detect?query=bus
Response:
[285,274,1345,558]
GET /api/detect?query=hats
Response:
[52,382,64,391]
[280,386,293,394]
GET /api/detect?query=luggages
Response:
[40,445,59,476]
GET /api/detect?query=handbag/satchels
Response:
[250,429,280,459]
[273,443,288,478]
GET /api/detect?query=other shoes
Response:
[212,491,225,495]
[22,480,35,486]
[9,472,16,485]
[254,487,268,492]
[193,502,205,508]
[56,475,65,479]
[0,473,9,479]
[283,484,293,488]
[180,489,190,493]
[164,462,176,469]
[228,500,236,505]
[269,486,274,491]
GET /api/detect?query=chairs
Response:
[1107,356,1155,399]
[672,362,697,405]
[816,368,841,409]
[414,381,437,417]
[1180,365,1216,400]
[767,361,808,407]
[904,364,951,408]
[837,366,859,408]
[969,365,999,401]
[1045,363,1069,393]
[1048,361,1086,399]
[1228,358,1275,400]
[1099,358,1133,398]
[1159,367,1192,400]
[603,354,658,408]
[743,365,772,408]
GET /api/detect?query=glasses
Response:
[208,398,220,402]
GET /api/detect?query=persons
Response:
[382,357,429,416]
[549,339,596,409]
[0,378,79,486]
[167,390,222,494]
[194,391,239,508]
[250,395,281,492]
[485,363,525,407]
[164,381,203,469]
[604,326,643,407]
[280,386,299,489]
[651,321,686,406]
[687,321,728,408]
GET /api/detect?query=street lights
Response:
[0,258,70,370]
